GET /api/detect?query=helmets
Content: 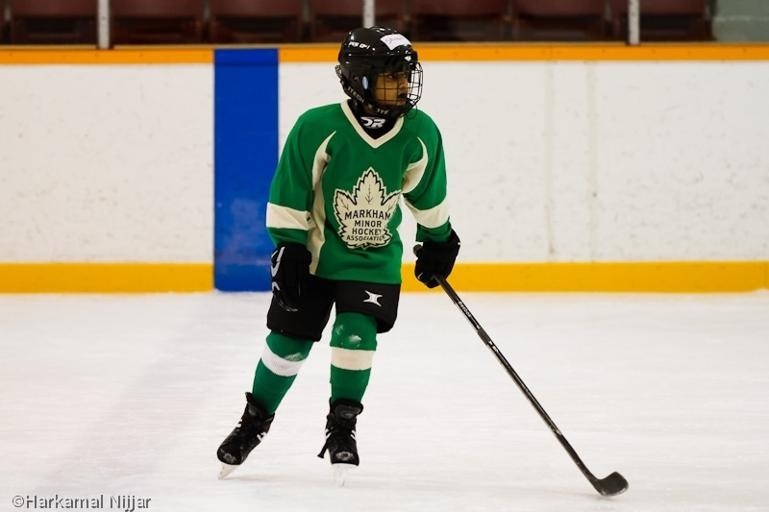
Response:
[336,25,424,121]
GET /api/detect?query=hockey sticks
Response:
[413,244,628,497]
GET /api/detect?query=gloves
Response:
[414,231,460,287]
[270,244,312,312]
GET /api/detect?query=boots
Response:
[218,393,274,463]
[326,398,363,464]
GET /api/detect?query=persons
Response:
[217,25,461,466]
